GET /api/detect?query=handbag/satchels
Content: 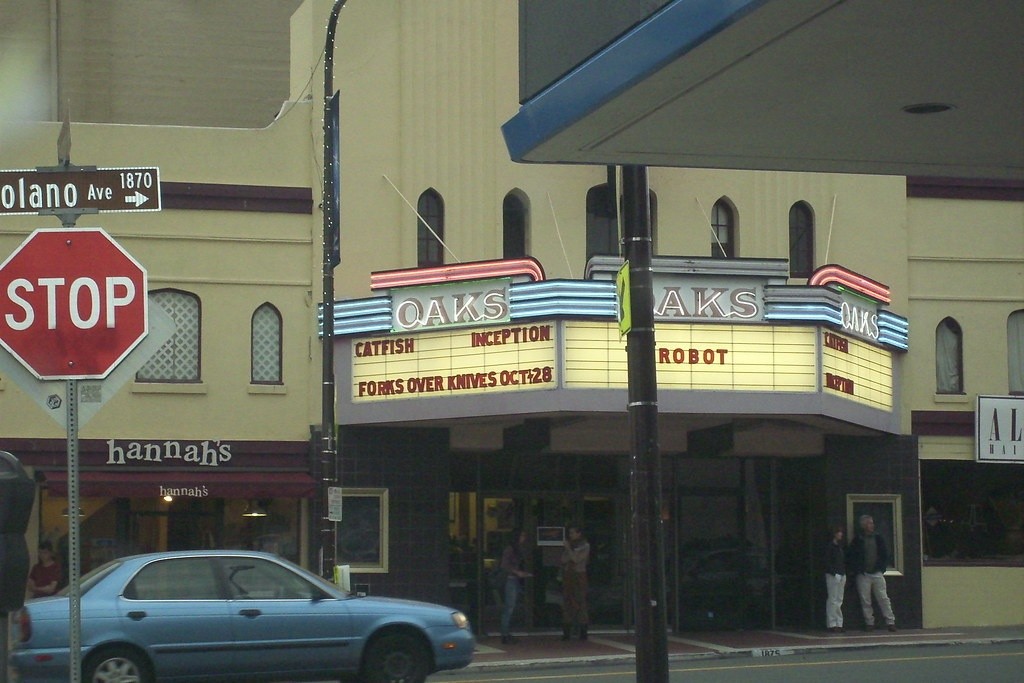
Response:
[487,568,507,589]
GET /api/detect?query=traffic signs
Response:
[1,166,163,214]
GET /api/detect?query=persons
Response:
[847,515,896,631]
[28,541,60,598]
[450,533,476,559]
[132,544,168,599]
[501,528,529,643]
[822,526,848,631]
[556,524,590,640]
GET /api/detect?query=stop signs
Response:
[0,227,151,385]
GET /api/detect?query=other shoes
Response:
[889,625,895,631]
[503,635,517,644]
[829,627,844,634]
[864,624,873,632]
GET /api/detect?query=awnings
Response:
[43,469,316,498]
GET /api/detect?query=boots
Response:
[561,622,569,641]
[579,624,587,640]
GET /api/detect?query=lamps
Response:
[63,507,86,518]
[242,498,267,518]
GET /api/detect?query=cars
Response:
[8,548,478,683]
